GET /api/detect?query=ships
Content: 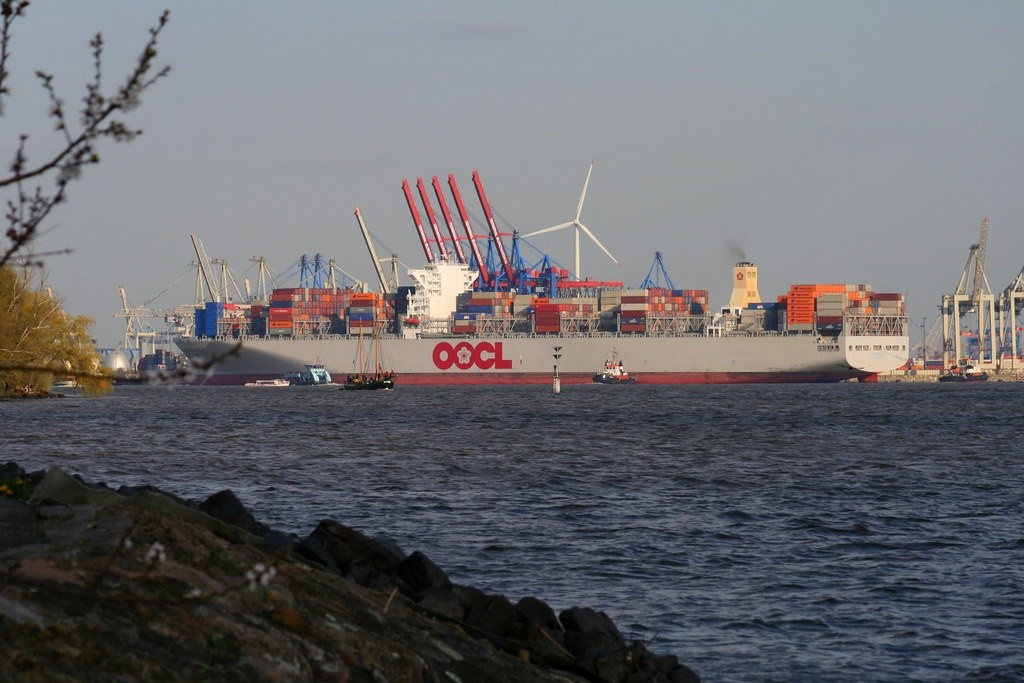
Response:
[173,172,909,383]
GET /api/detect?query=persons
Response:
[365,373,368,384]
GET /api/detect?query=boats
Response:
[938,336,988,382]
[245,379,291,387]
[592,347,637,384]
[282,357,331,385]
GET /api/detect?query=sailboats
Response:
[343,314,399,389]
